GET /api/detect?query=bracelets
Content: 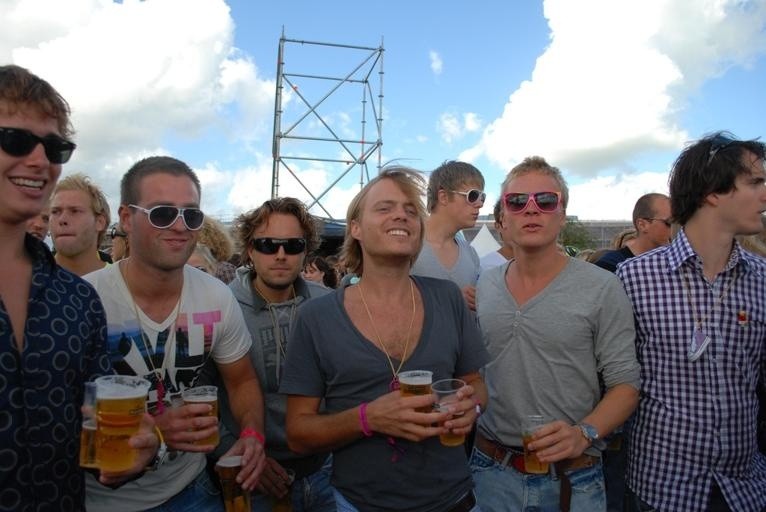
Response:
[359,403,373,436]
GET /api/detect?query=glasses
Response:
[127,206,206,231]
[250,236,308,257]
[0,127,78,165]
[706,130,738,167]
[450,188,488,206]
[642,216,673,228]
[111,228,127,238]
[502,191,566,214]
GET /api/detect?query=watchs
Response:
[145,425,170,471]
[573,422,599,448]
[475,399,481,418]
[240,429,264,445]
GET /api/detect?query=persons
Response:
[192,197,355,512]
[325,256,339,275]
[340,273,363,289]
[112,223,130,262]
[480,200,515,272]
[26,198,50,242]
[594,194,680,274]
[0,65,159,512]
[79,156,266,512]
[614,229,638,249]
[409,161,481,322]
[196,216,237,285]
[469,156,641,512]
[186,242,217,277]
[736,213,766,258]
[276,165,482,512]
[614,129,766,512]
[576,249,596,262]
[562,246,581,258]
[229,254,244,268]
[49,175,111,278]
[337,253,348,277]
[300,256,337,290]
[588,249,612,264]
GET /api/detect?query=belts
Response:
[471,431,598,476]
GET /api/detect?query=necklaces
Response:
[255,282,297,359]
[124,258,183,415]
[679,265,737,362]
[358,278,415,392]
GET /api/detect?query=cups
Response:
[94,375,152,472]
[78,381,100,469]
[431,377,470,447]
[519,415,550,477]
[397,370,433,431]
[215,454,252,512]
[182,384,220,449]
